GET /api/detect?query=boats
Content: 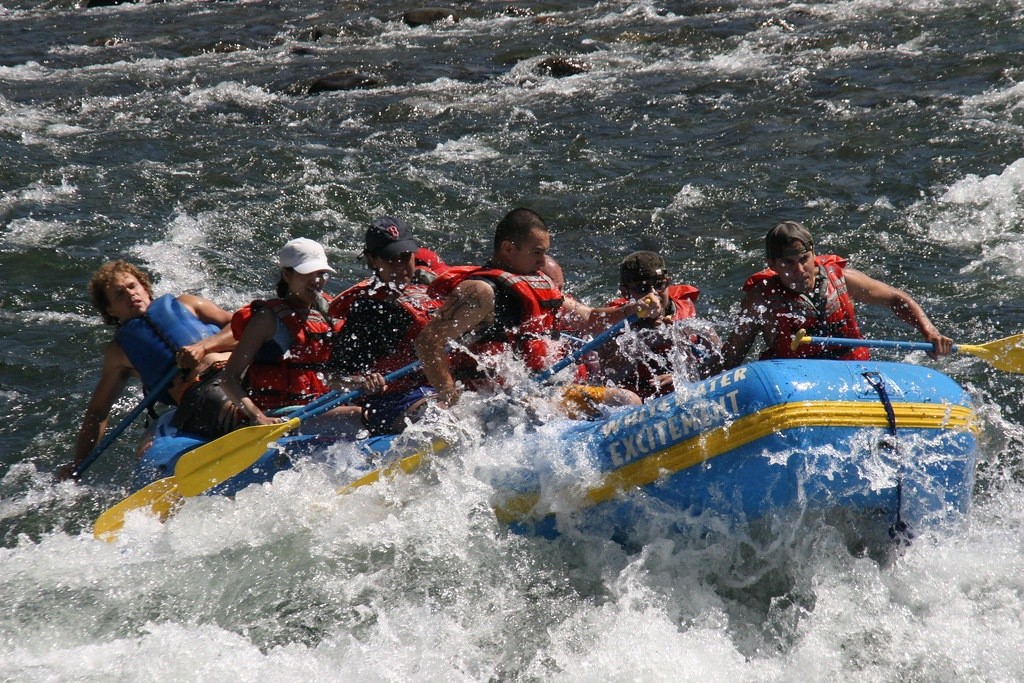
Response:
[130,356,981,563]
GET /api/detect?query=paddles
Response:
[174,328,453,497]
[786,328,1024,373]
[92,388,337,545]
[339,297,651,496]
[66,364,179,483]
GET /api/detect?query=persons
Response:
[719,222,952,373]
[57,208,720,487]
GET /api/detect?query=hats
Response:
[279,237,337,274]
[364,216,418,260]
[619,251,667,284]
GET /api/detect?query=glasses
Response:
[625,278,668,294]
